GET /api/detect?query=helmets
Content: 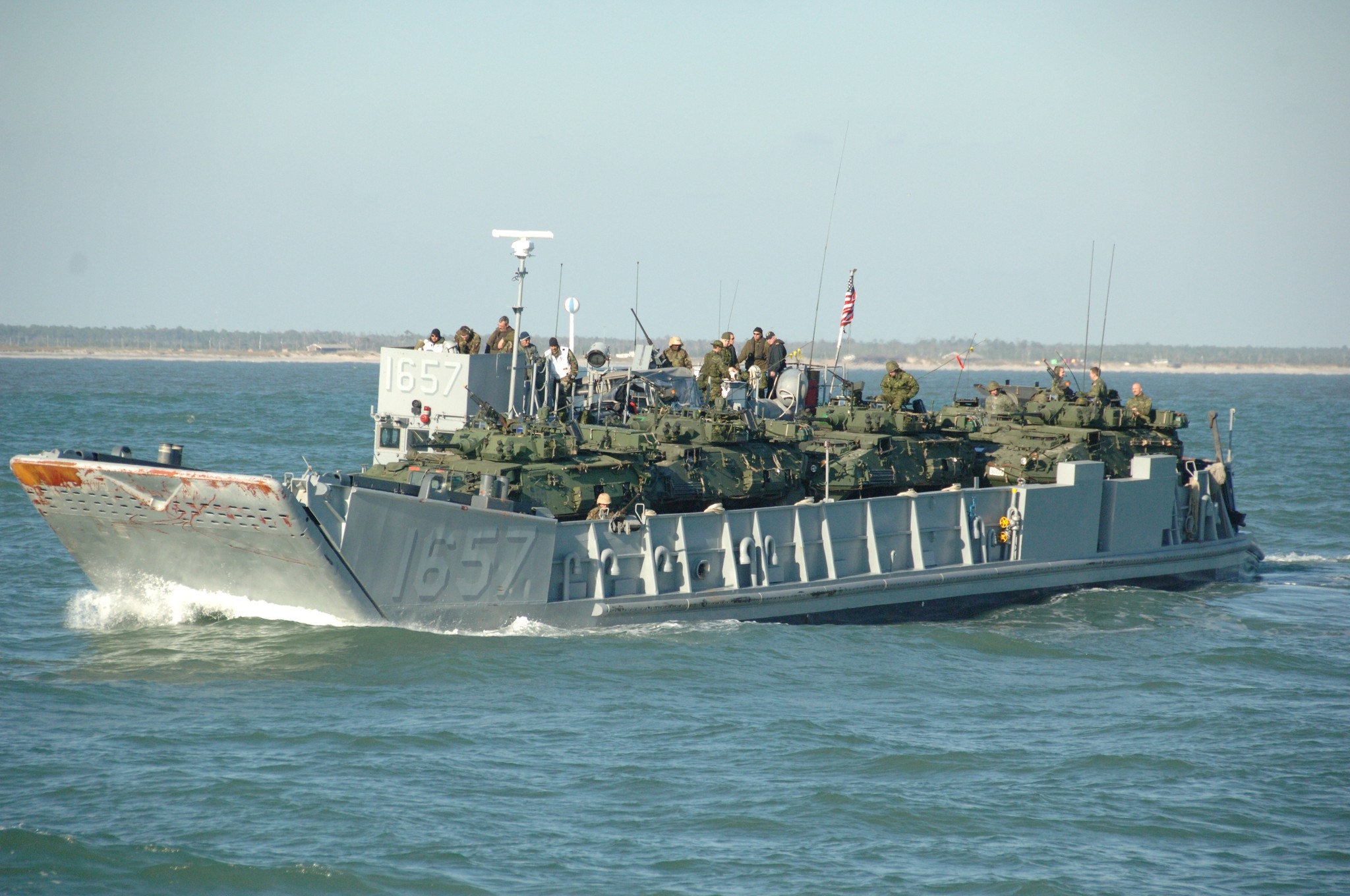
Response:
[987,380,999,391]
[596,493,611,504]
[668,336,682,346]
[886,361,899,373]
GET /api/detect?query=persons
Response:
[517,332,542,380]
[453,326,481,354]
[1125,383,1152,424]
[660,336,692,369]
[536,337,579,422]
[717,332,732,369]
[427,328,445,344]
[737,327,771,399]
[702,340,734,403]
[1087,367,1108,429]
[483,316,520,353]
[985,381,1017,411]
[874,361,919,413]
[766,332,787,400]
[728,332,740,371]
[1051,366,1071,401]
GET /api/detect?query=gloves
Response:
[568,373,577,380]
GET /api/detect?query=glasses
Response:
[731,338,734,340]
[431,335,438,337]
[753,333,761,335]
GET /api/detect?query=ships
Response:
[9,229,1251,635]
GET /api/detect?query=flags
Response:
[840,270,856,334]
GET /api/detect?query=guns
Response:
[462,384,518,434]
[1043,359,1080,404]
[828,367,865,407]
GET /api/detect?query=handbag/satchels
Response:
[697,375,705,389]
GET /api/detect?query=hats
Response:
[765,331,775,339]
[431,329,440,337]
[711,340,724,348]
[520,332,530,341]
[722,333,730,340]
[557,412,569,420]
[753,327,763,334]
[549,337,559,346]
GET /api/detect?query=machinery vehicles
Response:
[333,337,1193,523]
